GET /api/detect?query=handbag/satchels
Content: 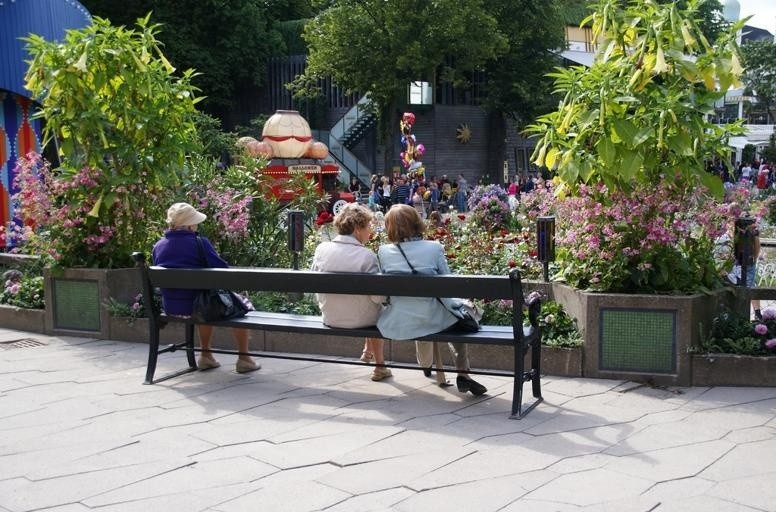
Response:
[192,286,256,321]
[448,309,479,335]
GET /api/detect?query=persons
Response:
[502,167,562,213]
[701,157,776,201]
[347,168,472,220]
[311,203,392,381]
[150,201,262,374]
[376,201,488,396]
[727,202,762,320]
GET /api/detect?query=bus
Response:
[263,164,356,220]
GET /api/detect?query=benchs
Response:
[131,251,544,420]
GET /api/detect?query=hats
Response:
[166,202,207,227]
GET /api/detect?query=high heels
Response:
[456,375,488,396]
[235,359,260,373]
[197,356,221,371]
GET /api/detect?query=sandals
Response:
[360,349,375,363]
[370,368,392,381]
